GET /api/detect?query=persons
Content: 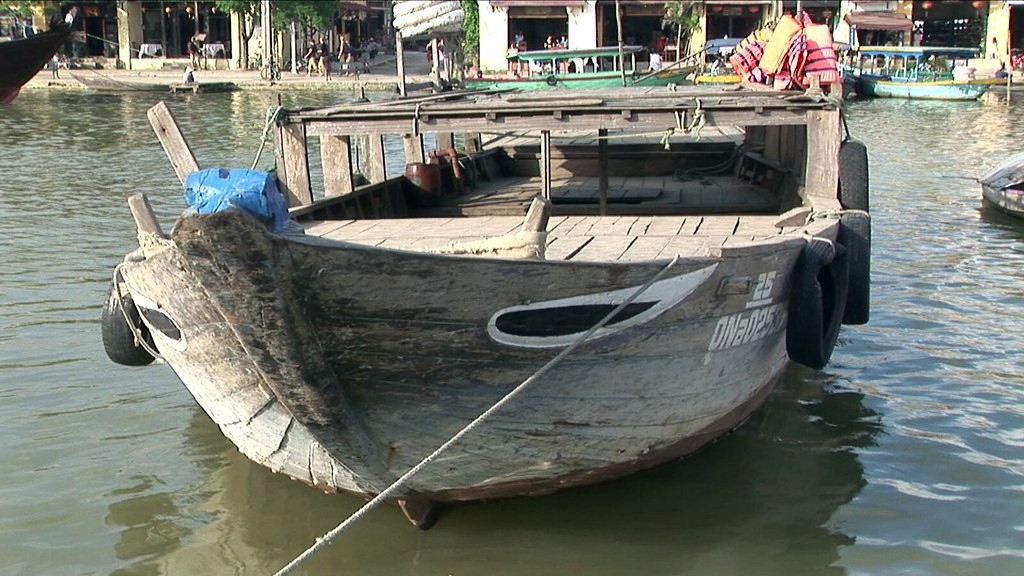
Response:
[51,52,60,79]
[509,32,568,74]
[183,67,195,84]
[304,36,381,83]
[187,37,200,68]
[426,39,436,74]
[650,36,684,70]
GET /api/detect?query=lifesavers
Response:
[547,76,557,86]
[839,212,872,327]
[837,141,870,212]
[785,239,849,368]
[102,281,161,368]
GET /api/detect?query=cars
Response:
[699,38,745,64]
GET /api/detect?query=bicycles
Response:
[260,56,282,81]
[283,53,314,74]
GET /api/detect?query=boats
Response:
[976,150,1024,221]
[101,37,873,518]
[452,47,697,95]
[848,69,1009,102]
[0,6,82,108]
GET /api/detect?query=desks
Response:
[203,44,226,59]
[138,43,164,59]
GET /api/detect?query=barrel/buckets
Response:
[405,162,441,210]
